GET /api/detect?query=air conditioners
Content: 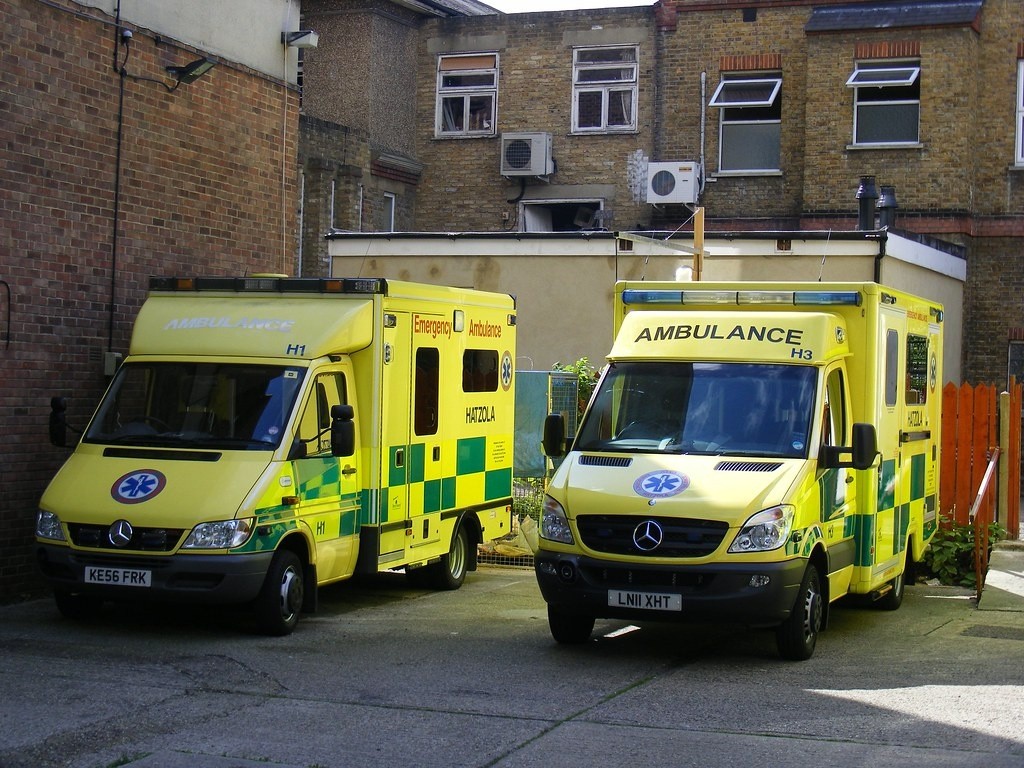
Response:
[500,131,555,184]
[647,161,701,216]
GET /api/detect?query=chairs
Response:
[169,375,220,436]
[614,394,680,445]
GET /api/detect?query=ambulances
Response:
[533,276,946,663]
[36,268,519,638]
